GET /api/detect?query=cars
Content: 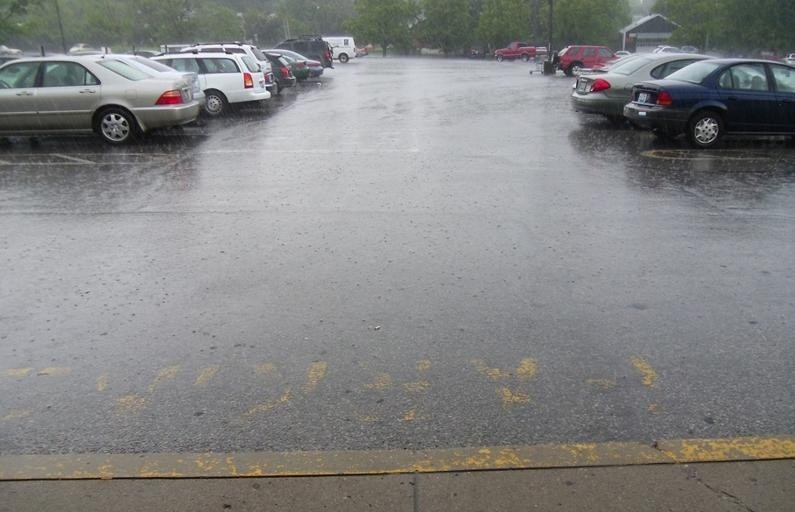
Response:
[552,45,794,150]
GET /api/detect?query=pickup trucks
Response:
[495,42,547,62]
